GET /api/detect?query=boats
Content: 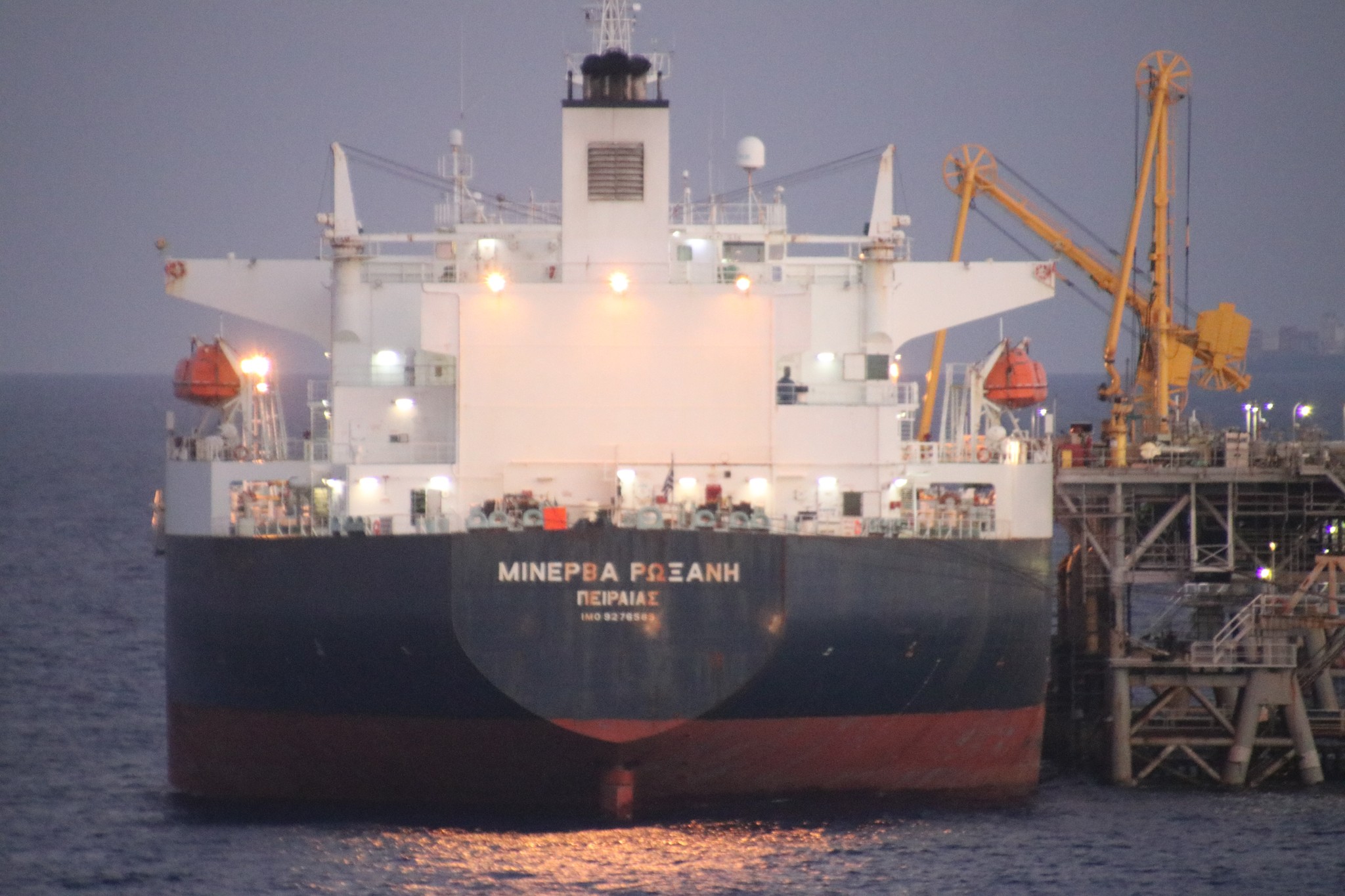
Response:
[982,349,1047,406]
[172,345,242,406]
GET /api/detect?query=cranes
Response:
[912,51,1253,464]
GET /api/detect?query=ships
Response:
[152,2,1054,800]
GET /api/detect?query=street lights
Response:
[1242,403,1274,445]
[1290,403,1311,475]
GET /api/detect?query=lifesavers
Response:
[234,445,249,462]
[940,493,962,512]
[977,448,993,462]
[1035,265,1050,279]
[373,519,380,535]
[169,261,186,278]
